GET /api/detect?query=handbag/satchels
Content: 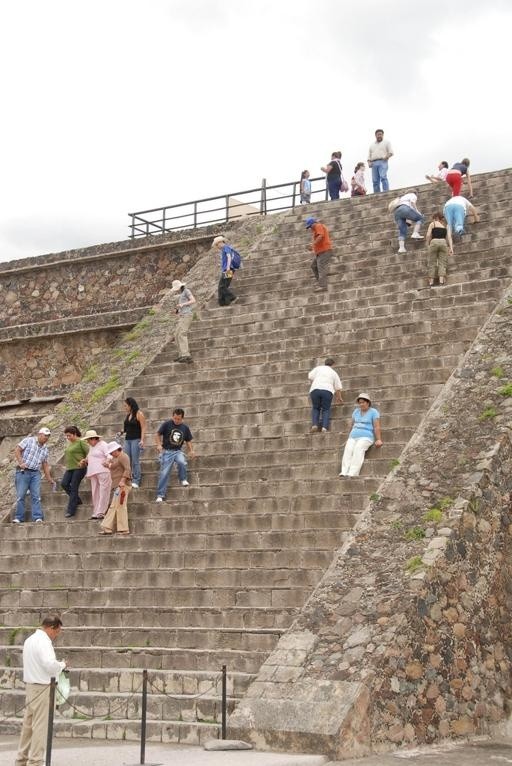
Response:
[340,180,348,192]
[388,196,400,214]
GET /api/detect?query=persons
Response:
[351,162,366,198]
[156,408,193,505]
[304,358,344,434]
[304,217,334,294]
[320,151,343,202]
[60,425,90,518]
[80,430,111,520]
[118,397,147,489]
[15,616,69,766]
[213,234,240,308]
[338,392,383,480]
[425,161,448,185]
[387,188,426,254]
[299,169,312,204]
[367,128,393,194]
[169,279,198,366]
[425,211,454,289]
[440,195,482,240]
[98,442,132,535]
[13,426,55,525]
[444,157,473,198]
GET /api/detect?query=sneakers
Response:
[321,427,327,432]
[398,248,406,252]
[411,232,424,239]
[339,472,347,477]
[231,297,239,304]
[181,480,189,486]
[132,482,139,489]
[35,519,43,523]
[314,286,328,293]
[310,425,318,433]
[88,513,130,535]
[156,497,163,503]
[174,357,180,361]
[13,518,21,524]
[178,356,192,362]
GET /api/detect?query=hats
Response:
[304,217,316,230]
[354,392,372,408]
[84,430,103,439]
[211,235,230,250]
[105,441,121,456]
[170,280,186,293]
[39,427,51,435]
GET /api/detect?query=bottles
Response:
[114,486,121,496]
[50,482,57,492]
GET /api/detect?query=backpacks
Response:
[226,245,241,270]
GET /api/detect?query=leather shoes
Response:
[65,513,72,518]
